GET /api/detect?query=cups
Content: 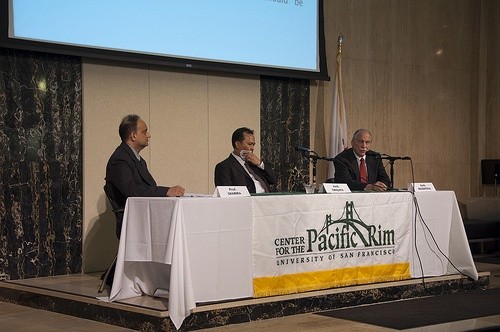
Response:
[304,185,315,193]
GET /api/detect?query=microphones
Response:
[294,145,310,152]
[366,151,383,156]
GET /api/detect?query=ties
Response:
[244,163,268,193]
[360,158,367,183]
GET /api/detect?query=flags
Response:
[329,53,349,178]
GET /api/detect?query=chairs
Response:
[100,185,125,292]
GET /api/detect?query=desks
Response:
[105,192,478,332]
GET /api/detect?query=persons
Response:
[104,113,185,242]
[332,128,391,193]
[214,128,278,195]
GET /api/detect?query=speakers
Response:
[480,159,500,185]
